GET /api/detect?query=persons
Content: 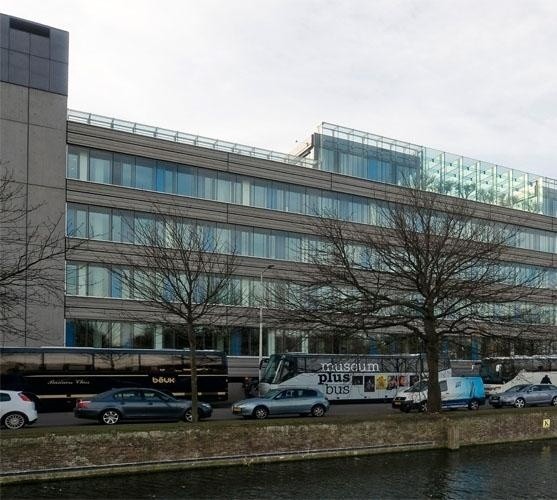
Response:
[540,375,552,384]
[399,376,406,387]
[241,376,257,397]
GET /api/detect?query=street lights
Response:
[258,264,275,397]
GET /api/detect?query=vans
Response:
[391,376,486,413]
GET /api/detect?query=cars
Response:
[231,385,330,419]
[0,390,39,430]
[489,383,557,408]
[73,387,213,425]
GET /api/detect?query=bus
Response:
[474,355,557,399]
[258,352,453,400]
[0,346,228,410]
